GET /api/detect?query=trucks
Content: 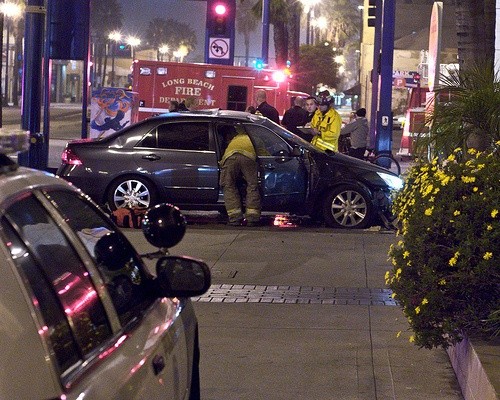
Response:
[128,57,315,127]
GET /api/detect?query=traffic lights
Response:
[215,2,226,35]
[367,0,377,27]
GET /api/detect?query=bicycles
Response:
[338,137,402,178]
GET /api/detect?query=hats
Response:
[356,108,366,116]
[314,90,334,104]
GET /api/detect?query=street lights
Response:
[128,36,139,65]
[108,30,121,86]
[0,1,14,106]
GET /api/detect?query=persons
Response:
[220,132,271,227]
[169,89,369,160]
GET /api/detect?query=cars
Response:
[55,107,406,230]
[0,150,212,400]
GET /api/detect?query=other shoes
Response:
[247,217,265,226]
[228,220,242,225]
